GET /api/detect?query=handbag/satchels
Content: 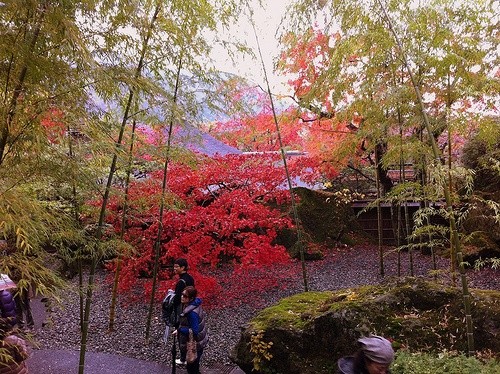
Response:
[15,344,30,361]
[186,328,198,363]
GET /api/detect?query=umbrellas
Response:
[172,331,176,374]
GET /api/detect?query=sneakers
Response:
[175,359,187,364]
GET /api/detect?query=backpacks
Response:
[161,289,179,326]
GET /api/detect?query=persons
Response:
[0,234,34,374]
[328,336,394,374]
[172,286,209,374]
[168,258,194,364]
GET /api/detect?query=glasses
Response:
[181,292,191,298]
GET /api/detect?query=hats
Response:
[358,334,394,364]
